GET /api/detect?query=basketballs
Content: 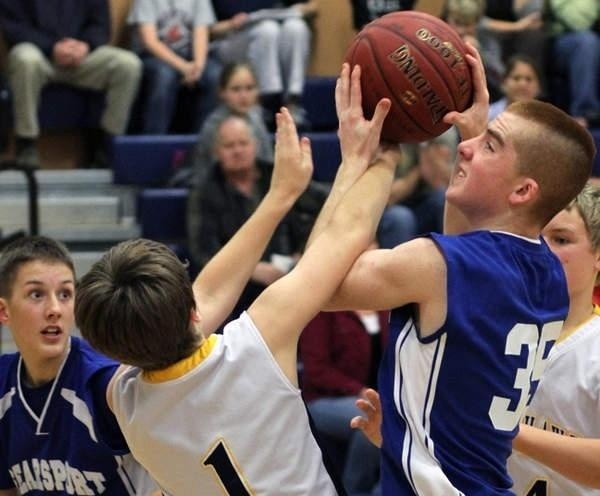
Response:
[345,11,474,142]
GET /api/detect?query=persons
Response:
[479,0,570,108]
[208,0,318,128]
[123,1,225,138]
[307,39,596,496]
[548,0,600,114]
[188,116,314,310]
[436,1,505,85]
[351,0,417,32]
[352,179,600,496]
[74,106,402,496]
[2,1,143,169]
[484,57,539,121]
[0,236,134,496]
[194,59,273,182]
[298,308,392,496]
